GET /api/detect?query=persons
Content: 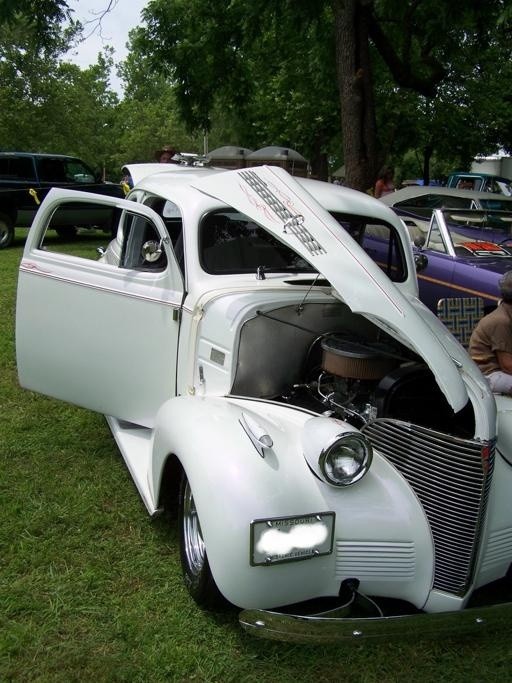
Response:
[374,163,396,199]
[466,266,512,398]
[159,144,177,162]
